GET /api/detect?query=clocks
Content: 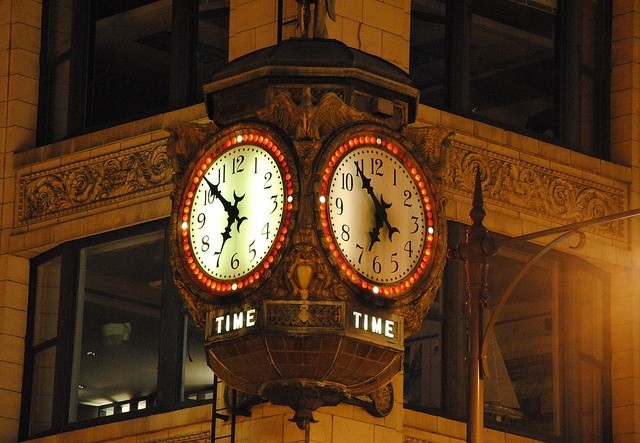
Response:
[169,119,299,304]
[311,121,446,305]
